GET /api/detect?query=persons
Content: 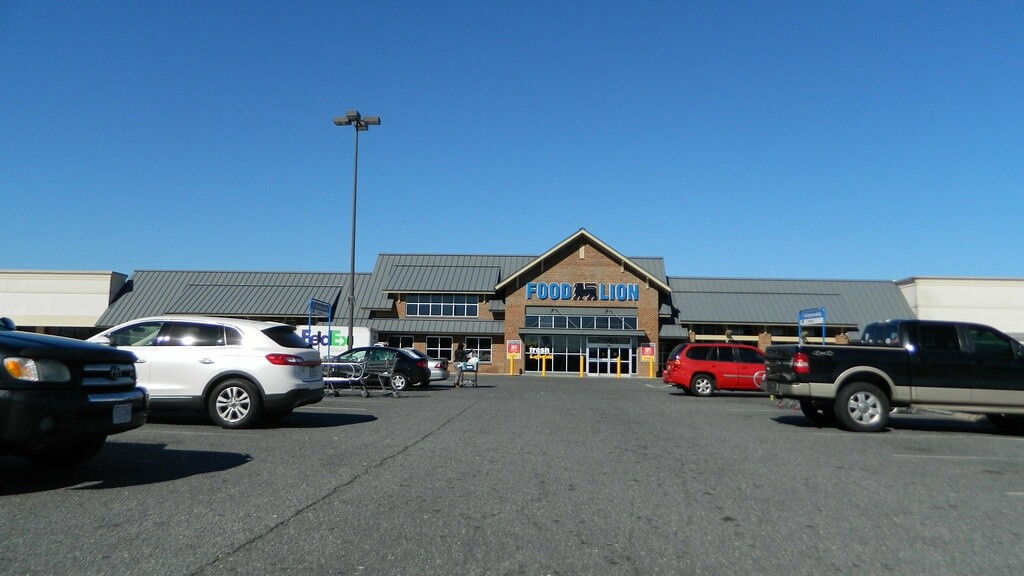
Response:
[452,343,473,388]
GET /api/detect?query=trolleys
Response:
[459,359,478,388]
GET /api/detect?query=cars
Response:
[402,347,449,382]
[662,342,771,396]
[0,329,150,463]
[81,313,325,429]
[317,345,432,392]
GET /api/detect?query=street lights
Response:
[333,109,382,349]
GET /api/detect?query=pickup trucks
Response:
[763,318,1024,431]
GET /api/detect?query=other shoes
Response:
[455,383,460,388]
